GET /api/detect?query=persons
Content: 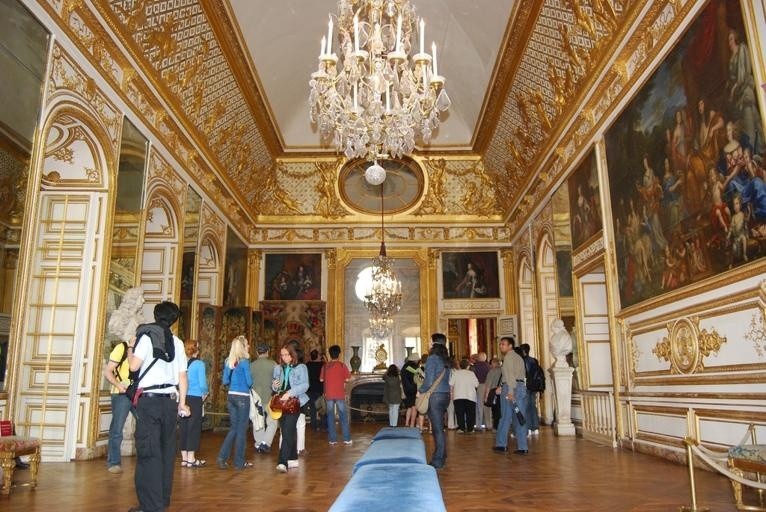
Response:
[400,352,419,428]
[739,147,765,180]
[413,354,433,433]
[686,99,724,200]
[289,265,312,300]
[721,122,766,217]
[127,301,188,512]
[615,218,625,287]
[306,349,326,433]
[249,343,279,455]
[724,195,752,268]
[484,357,503,433]
[320,344,353,445]
[108,287,146,341]
[623,197,655,281]
[272,270,289,300]
[521,344,539,438]
[664,128,672,157]
[104,323,147,474]
[452,358,481,434]
[659,238,676,289]
[454,263,487,299]
[575,185,589,231]
[636,155,666,245]
[491,338,531,455]
[469,354,478,370]
[670,230,687,281]
[446,357,459,430]
[690,242,706,273]
[420,344,451,469]
[658,157,684,221]
[383,364,403,428]
[707,169,731,231]
[179,339,210,468]
[280,276,287,298]
[216,335,254,469]
[722,28,764,153]
[549,318,572,356]
[271,344,310,473]
[496,347,530,439]
[431,334,446,345]
[473,352,492,432]
[669,110,693,167]
[624,212,652,280]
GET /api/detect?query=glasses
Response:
[280,354,291,357]
[197,346,200,349]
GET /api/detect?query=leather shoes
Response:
[492,446,508,454]
[514,449,528,455]
[428,462,445,470]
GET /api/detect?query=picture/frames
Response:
[441,251,501,300]
[568,146,603,256]
[603,0,766,314]
[263,252,322,301]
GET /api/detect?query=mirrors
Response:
[339,246,431,370]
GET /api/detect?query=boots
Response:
[15,457,30,470]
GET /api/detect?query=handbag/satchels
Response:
[484,372,502,407]
[125,357,159,401]
[269,394,301,414]
[415,368,447,414]
[267,396,283,420]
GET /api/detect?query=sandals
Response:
[187,459,206,468]
[180,460,187,468]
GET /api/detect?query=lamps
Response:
[308,0,451,186]
[363,184,403,338]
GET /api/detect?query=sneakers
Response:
[287,459,299,468]
[217,455,229,469]
[328,441,338,445]
[234,461,253,468]
[456,429,464,433]
[108,464,123,474]
[275,463,287,473]
[298,449,311,456]
[257,448,262,453]
[343,440,353,444]
[532,429,540,435]
[260,443,271,453]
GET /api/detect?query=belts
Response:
[515,379,524,383]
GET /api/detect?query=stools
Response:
[726,443,766,512]
[0,435,40,498]
